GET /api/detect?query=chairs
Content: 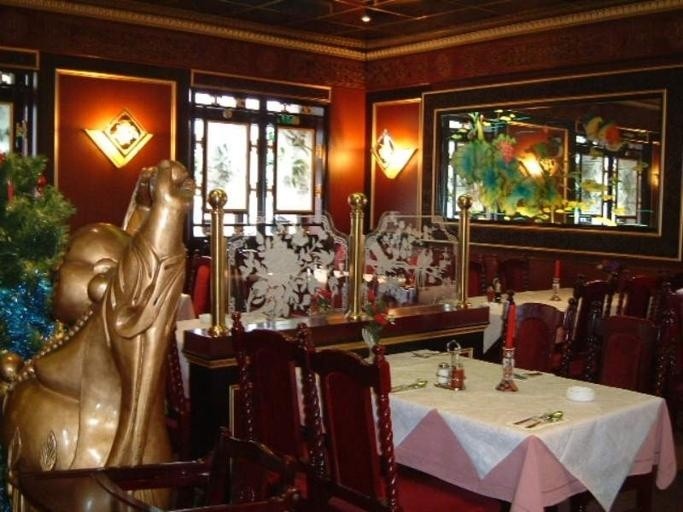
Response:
[6,428,300,510]
[226,326,310,511]
[1,240,682,380]
[295,345,502,512]
[580,312,676,398]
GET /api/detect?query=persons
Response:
[0,156,199,511]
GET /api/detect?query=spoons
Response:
[524,411,565,428]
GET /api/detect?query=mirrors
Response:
[417,69,683,262]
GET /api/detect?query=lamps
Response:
[82,111,154,167]
[370,128,417,179]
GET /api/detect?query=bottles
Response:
[438,362,463,388]
[486,283,502,301]
